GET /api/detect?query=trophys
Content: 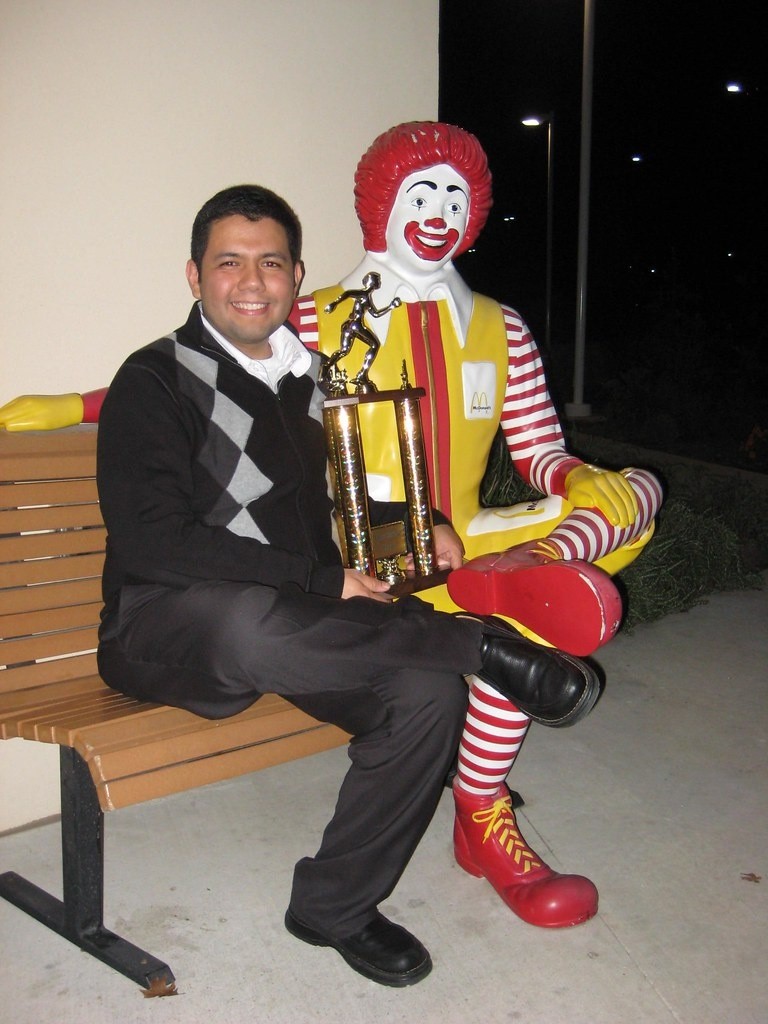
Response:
[317,273,454,596]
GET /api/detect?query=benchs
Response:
[0,422,355,990]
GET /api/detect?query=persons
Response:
[0,114,663,927]
[96,184,602,988]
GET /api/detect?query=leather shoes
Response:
[454,612,601,730]
[284,906,433,987]
